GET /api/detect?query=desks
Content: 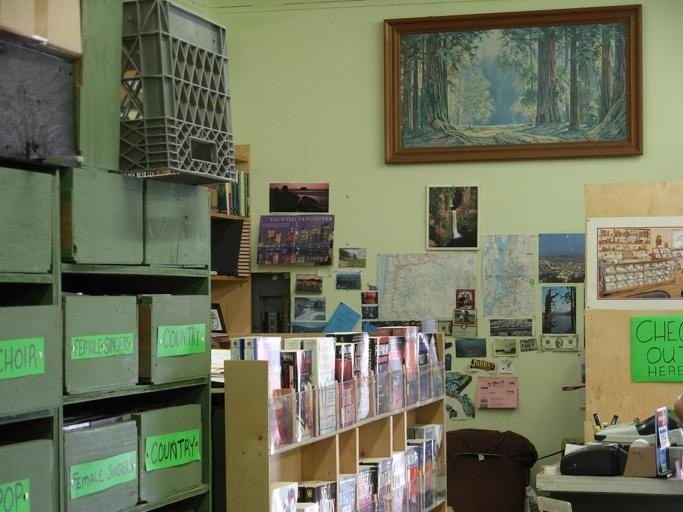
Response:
[535,441,683,511]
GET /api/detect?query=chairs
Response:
[446,428,539,512]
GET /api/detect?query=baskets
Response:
[119,1,239,186]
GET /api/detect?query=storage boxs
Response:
[60,168,144,267]
[66,296,139,394]
[63,420,139,512]
[146,178,212,268]
[140,296,215,386]
[0,438,61,512]
[0,166,55,274]
[0,306,64,416]
[139,403,206,503]
[0,0,83,55]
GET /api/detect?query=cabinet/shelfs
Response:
[224,314,453,512]
[208,140,253,392]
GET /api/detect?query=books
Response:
[269,425,447,512]
[230,326,443,455]
[210,170,249,217]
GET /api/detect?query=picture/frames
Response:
[211,303,227,333]
[426,184,481,251]
[384,4,646,162]
[584,217,683,311]
